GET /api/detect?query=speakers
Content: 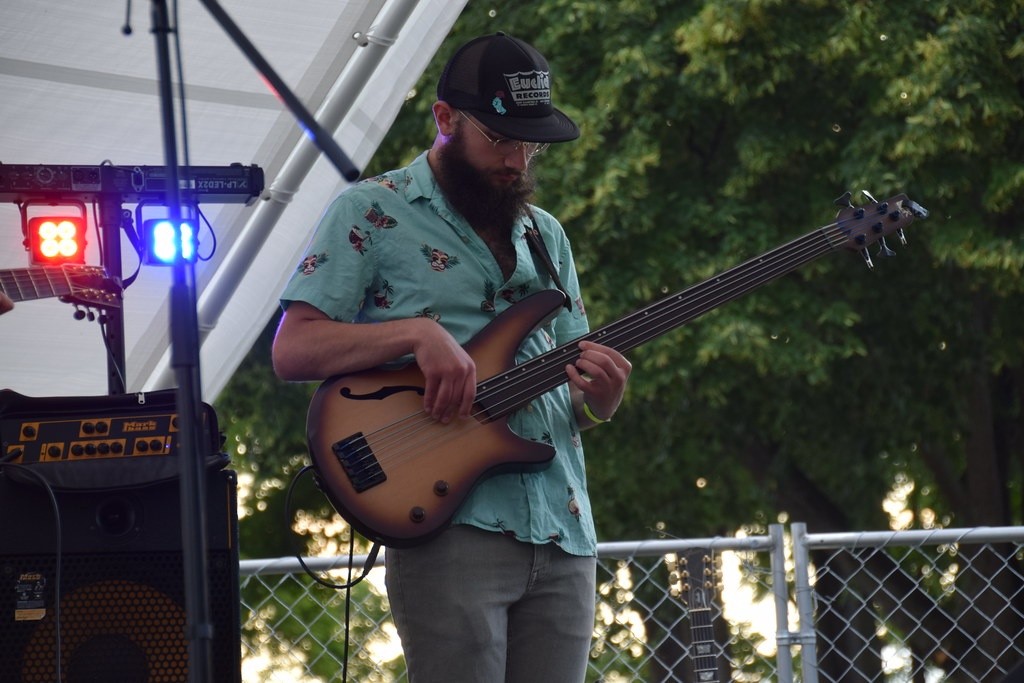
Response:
[0,468,242,683]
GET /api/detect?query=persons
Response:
[272,30,632,683]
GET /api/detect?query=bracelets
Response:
[584,404,610,423]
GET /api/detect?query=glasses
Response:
[456,109,550,157]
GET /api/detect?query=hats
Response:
[436,30,581,144]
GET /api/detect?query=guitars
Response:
[0,264,126,324]
[304,186,932,552]
